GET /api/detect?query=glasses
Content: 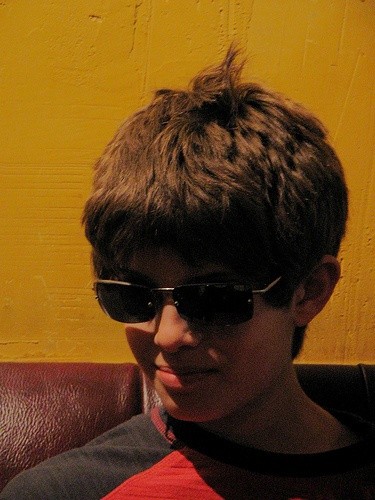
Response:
[92,266,284,329]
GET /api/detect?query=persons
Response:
[1,58,374,500]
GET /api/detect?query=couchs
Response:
[0,362,375,500]
